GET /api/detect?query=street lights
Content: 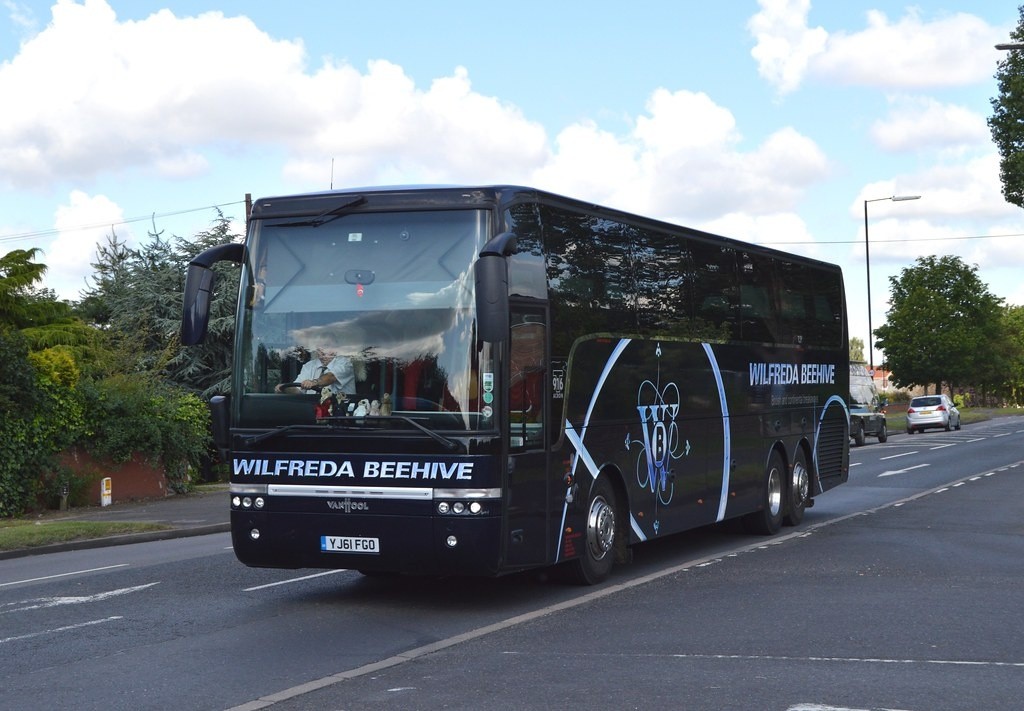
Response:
[864,195,921,381]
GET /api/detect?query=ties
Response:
[316,367,327,394]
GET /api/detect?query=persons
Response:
[274,332,358,417]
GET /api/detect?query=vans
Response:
[849,360,890,445]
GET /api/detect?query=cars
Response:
[906,394,961,434]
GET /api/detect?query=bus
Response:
[179,183,849,588]
